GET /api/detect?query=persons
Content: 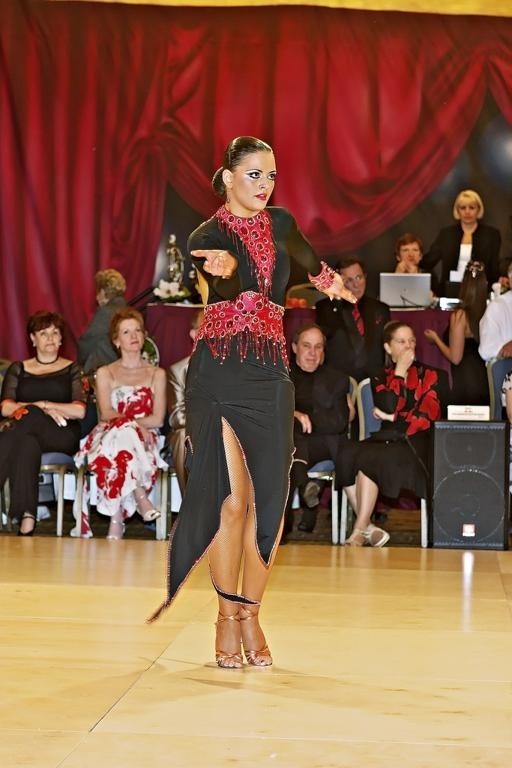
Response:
[77,270,127,434]
[334,320,446,547]
[414,188,500,298]
[424,256,511,404]
[314,257,392,384]
[389,233,424,274]
[168,308,206,498]
[1,312,85,538]
[74,312,169,540]
[142,135,359,672]
[285,323,355,510]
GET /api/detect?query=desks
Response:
[147,305,455,379]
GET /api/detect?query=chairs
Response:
[285,282,332,309]
[485,358,512,420]
[1,339,434,547]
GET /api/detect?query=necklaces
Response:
[35,354,59,365]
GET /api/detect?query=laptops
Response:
[379,272,432,308]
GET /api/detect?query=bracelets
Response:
[43,400,48,409]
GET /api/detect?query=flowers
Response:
[154,279,184,302]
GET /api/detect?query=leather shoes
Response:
[301,480,320,510]
[299,513,317,531]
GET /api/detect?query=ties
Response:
[352,302,365,336]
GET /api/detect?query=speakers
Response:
[434,421,506,550]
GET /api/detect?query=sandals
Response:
[18,513,37,536]
[344,523,390,548]
[107,519,125,540]
[214,603,273,669]
[137,494,161,522]
